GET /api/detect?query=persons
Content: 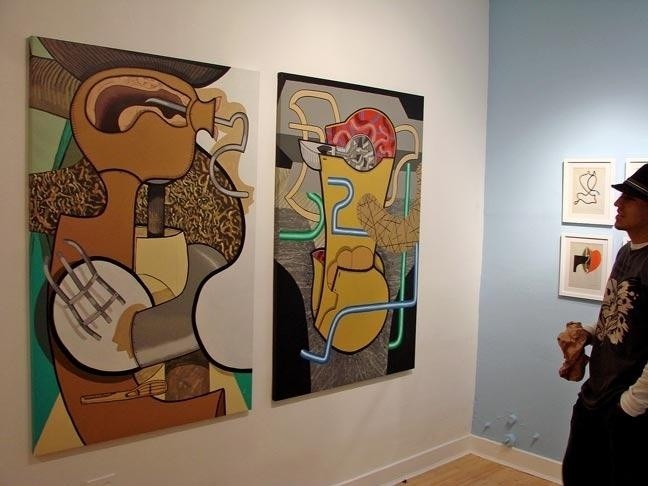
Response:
[555,161,647,485]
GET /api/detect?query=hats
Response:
[611,163,648,201]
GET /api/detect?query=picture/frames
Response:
[562,157,616,227]
[624,159,648,182]
[558,232,614,303]
